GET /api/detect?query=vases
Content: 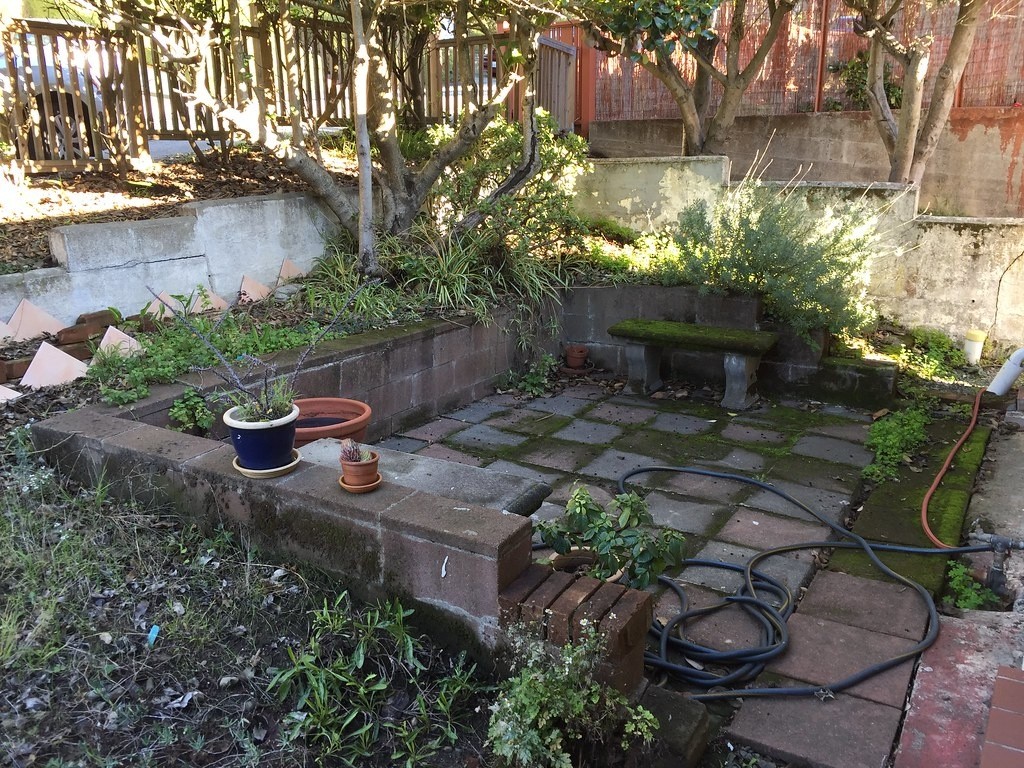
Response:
[293,398,371,448]
[564,344,590,368]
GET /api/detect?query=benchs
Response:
[606,318,783,410]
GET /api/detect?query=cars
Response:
[0,16,117,160]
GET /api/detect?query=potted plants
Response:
[531,479,688,592]
[218,372,300,470]
[339,438,379,486]
